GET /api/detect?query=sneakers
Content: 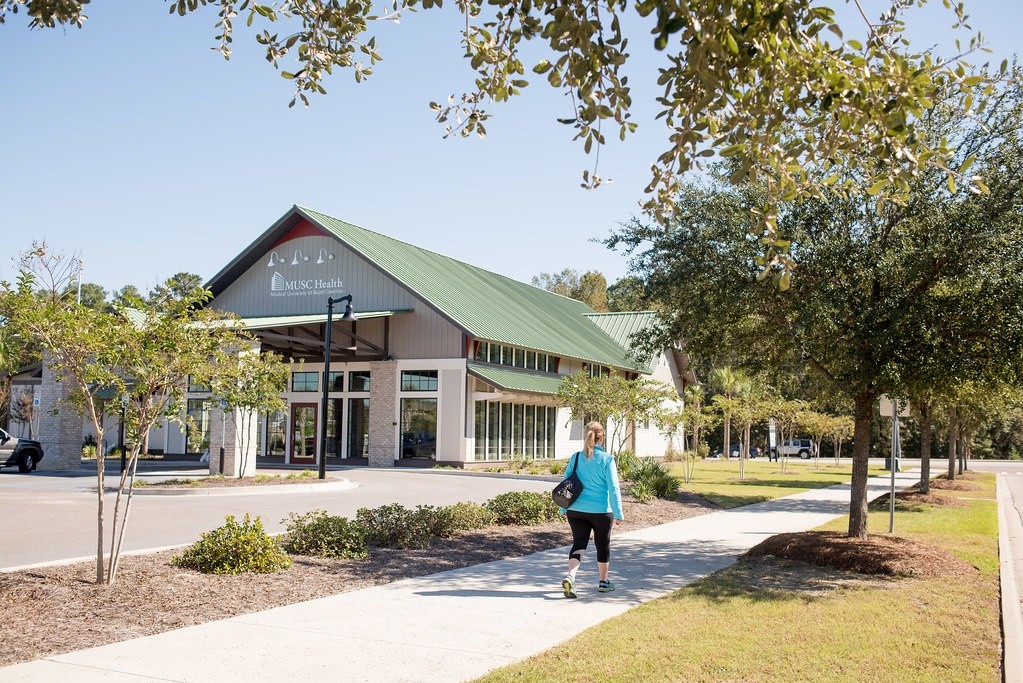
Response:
[561,574,577,599]
[599,579,616,591]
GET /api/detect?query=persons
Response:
[557,421,624,599]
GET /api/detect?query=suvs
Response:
[771,439,818,459]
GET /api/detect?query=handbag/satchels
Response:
[552,452,584,509]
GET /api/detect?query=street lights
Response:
[319,293,360,480]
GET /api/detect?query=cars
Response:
[0,428,44,474]
[402,430,436,461]
[713,444,758,459]
